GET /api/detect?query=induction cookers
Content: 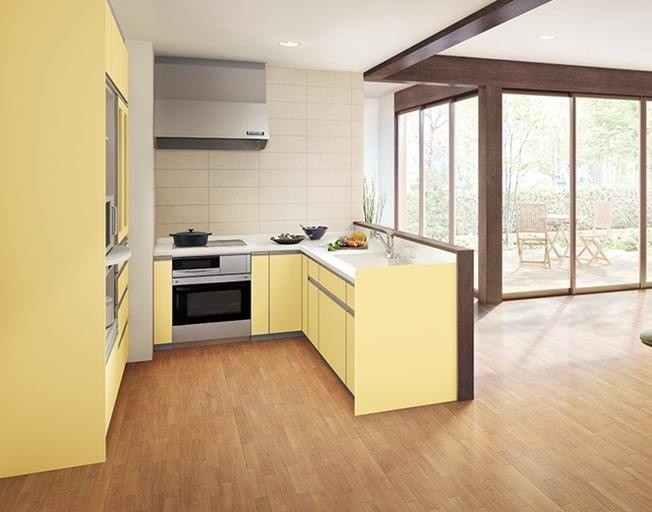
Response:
[171,240,247,248]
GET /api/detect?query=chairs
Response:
[577,200,613,267]
[514,201,551,269]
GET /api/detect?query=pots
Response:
[168,229,213,247]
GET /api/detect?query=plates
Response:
[270,235,305,245]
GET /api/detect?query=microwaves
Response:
[105,196,118,255]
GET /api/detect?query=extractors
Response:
[153,55,269,151]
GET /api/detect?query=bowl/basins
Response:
[300,224,328,241]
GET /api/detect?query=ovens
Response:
[171,255,251,342]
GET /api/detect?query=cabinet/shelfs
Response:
[250,254,355,399]
[105,96,131,439]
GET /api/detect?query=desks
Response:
[535,214,586,265]
